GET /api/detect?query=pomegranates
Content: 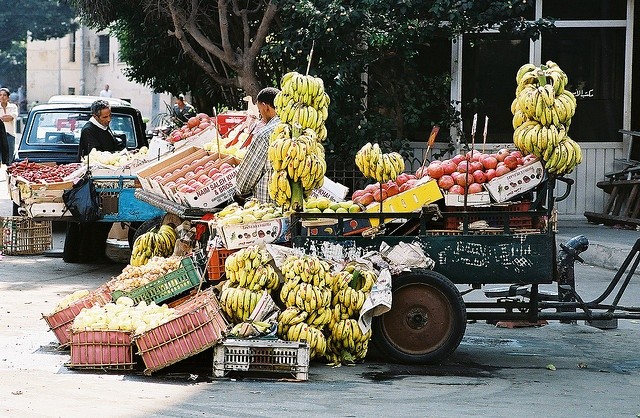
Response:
[418,148,534,194]
[351,174,416,205]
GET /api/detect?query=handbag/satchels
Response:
[60,172,104,232]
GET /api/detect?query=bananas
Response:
[219,200,280,224]
[511,59,582,174]
[267,71,330,204]
[279,254,334,359]
[126,225,177,266]
[218,245,280,324]
[326,264,378,367]
[355,142,404,181]
[230,320,271,337]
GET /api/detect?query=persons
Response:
[99,84,113,99]
[173,93,197,122]
[7,90,19,103]
[234,87,279,207]
[77,99,127,161]
[0,88,19,165]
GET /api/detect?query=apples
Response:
[155,154,234,194]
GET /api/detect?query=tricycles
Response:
[291,170,639,364]
[89,176,167,250]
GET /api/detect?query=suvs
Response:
[11,102,152,229]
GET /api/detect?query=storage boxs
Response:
[365,178,443,228]
[481,161,549,204]
[136,145,242,208]
[217,217,290,251]
[5,171,75,216]
[444,190,490,207]
[306,217,371,236]
